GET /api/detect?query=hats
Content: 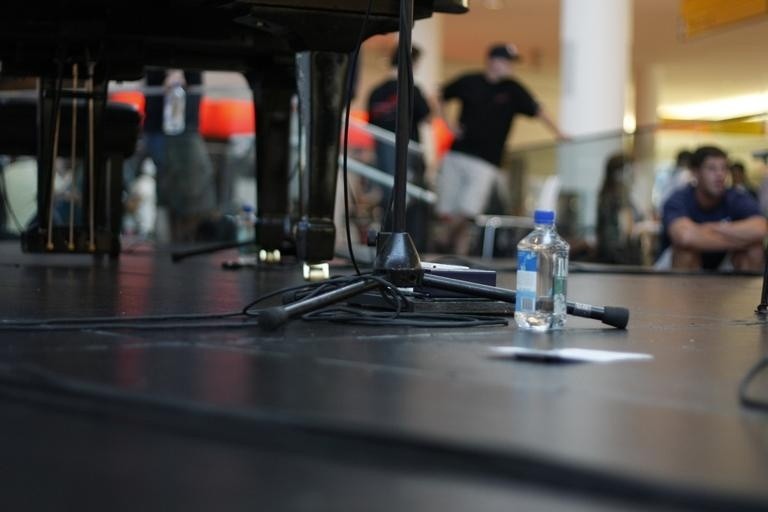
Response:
[488,43,522,61]
[391,45,420,67]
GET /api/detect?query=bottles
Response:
[236,205,257,242]
[513,208,570,333]
[164,81,187,135]
[129,160,158,233]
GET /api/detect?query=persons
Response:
[429,39,574,255]
[592,142,766,275]
[369,44,438,260]
